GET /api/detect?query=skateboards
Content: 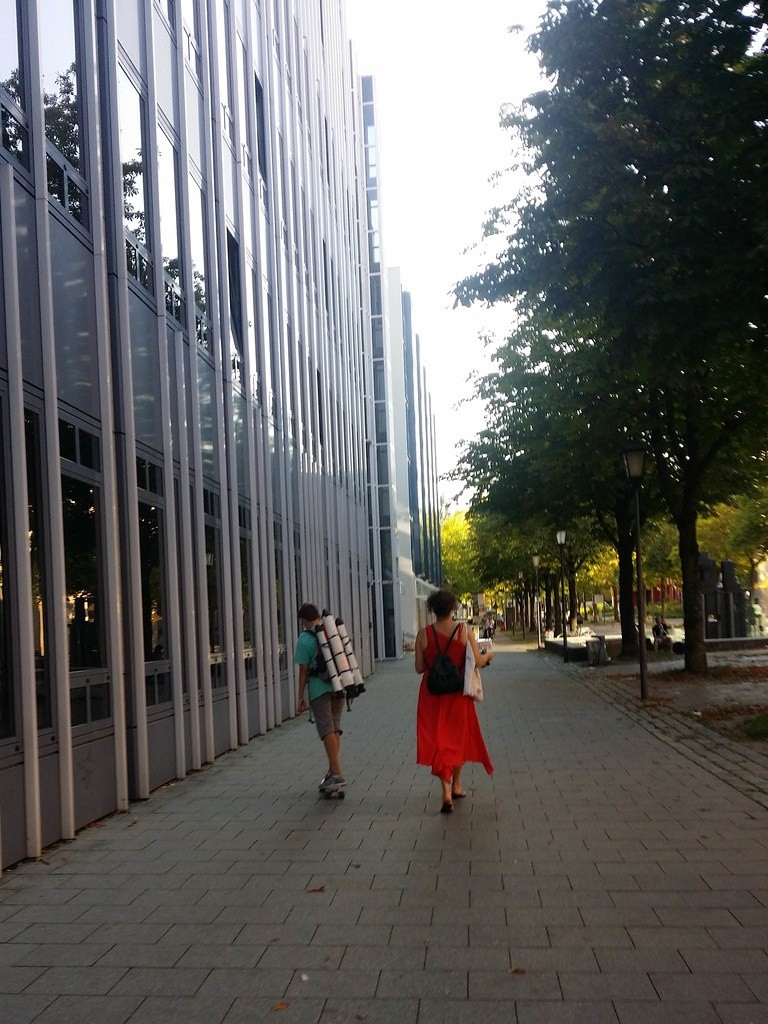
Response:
[320,776,348,799]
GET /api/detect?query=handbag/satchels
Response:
[464,624,483,702]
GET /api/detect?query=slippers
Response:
[453,790,467,798]
[440,802,453,813]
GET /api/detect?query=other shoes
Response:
[318,775,346,789]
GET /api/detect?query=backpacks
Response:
[425,623,466,695]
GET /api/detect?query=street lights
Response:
[621,441,649,699]
[532,551,542,650]
[555,528,569,662]
[517,569,525,640]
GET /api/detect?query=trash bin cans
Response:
[586,635,611,665]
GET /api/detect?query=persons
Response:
[413,588,494,814]
[465,614,497,639]
[293,602,347,792]
[652,614,676,658]
